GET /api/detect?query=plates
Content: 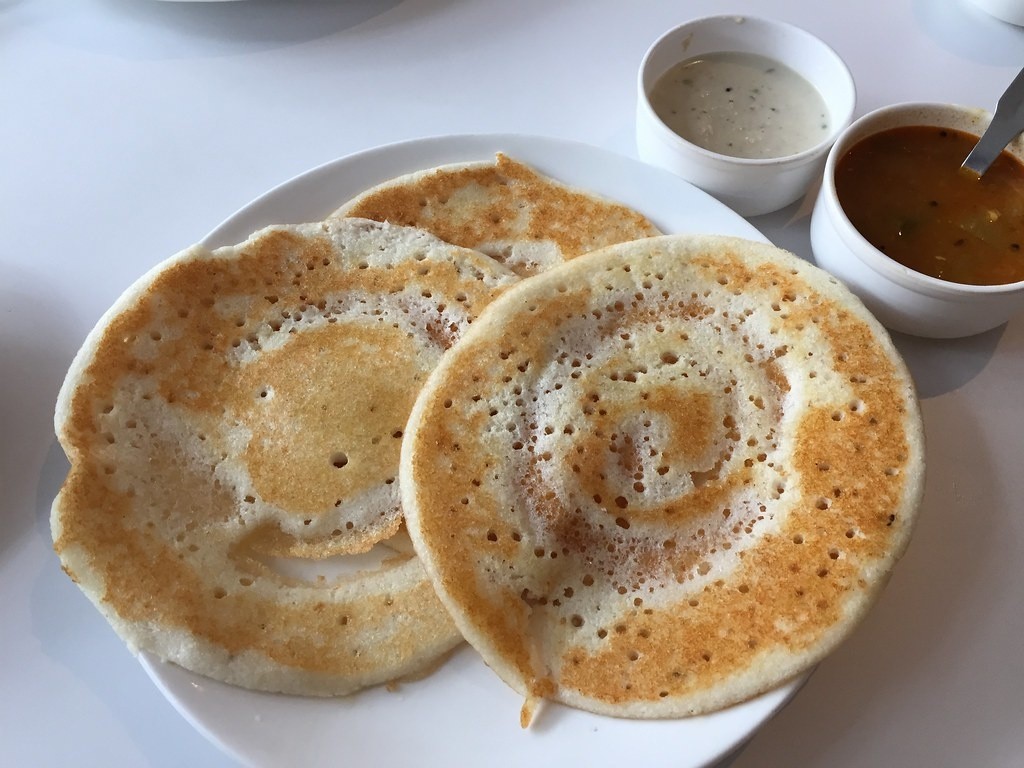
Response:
[128,131,819,768]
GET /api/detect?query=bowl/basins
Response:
[975,0,1024,27]
[807,97,1024,338]
[636,14,857,216]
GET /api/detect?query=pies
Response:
[49,215,524,698]
[398,232,924,726]
[322,152,665,282]
